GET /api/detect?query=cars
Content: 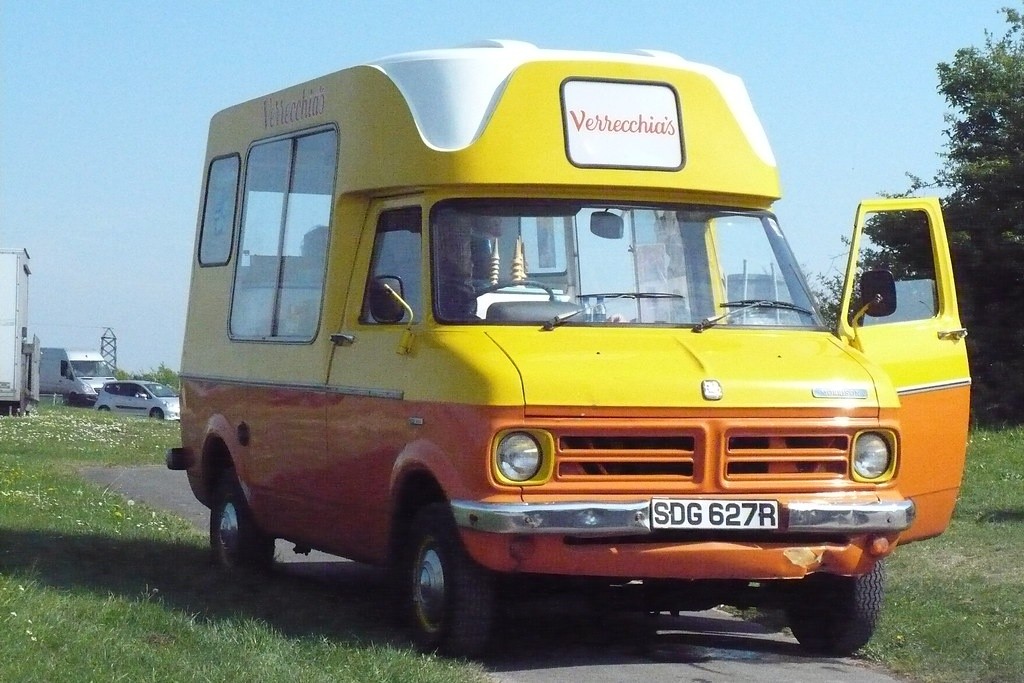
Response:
[95,380,180,422]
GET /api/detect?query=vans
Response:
[165,39,972,659]
[38,347,118,408]
